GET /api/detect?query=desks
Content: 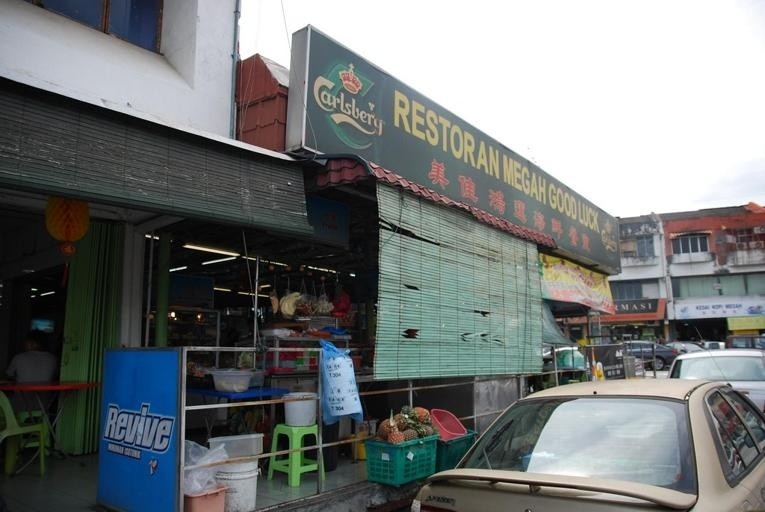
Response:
[0,382,98,475]
[187,387,289,448]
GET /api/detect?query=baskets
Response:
[364,435,440,486]
[297,314,356,329]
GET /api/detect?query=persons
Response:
[8,332,65,407]
[655,335,666,345]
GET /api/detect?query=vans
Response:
[726,334,765,349]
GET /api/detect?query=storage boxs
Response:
[207,433,264,459]
[184,485,229,512]
[437,429,479,472]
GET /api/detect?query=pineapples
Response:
[373,405,438,444]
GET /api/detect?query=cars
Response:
[412,378,764,512]
[669,348,764,413]
[622,340,678,371]
[667,342,704,354]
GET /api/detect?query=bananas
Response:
[279,292,302,319]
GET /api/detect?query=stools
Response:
[266,423,325,488]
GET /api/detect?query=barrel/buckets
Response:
[216,469,259,512]
[312,420,339,472]
[284,392,317,427]
[356,420,377,436]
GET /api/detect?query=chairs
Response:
[0,392,49,479]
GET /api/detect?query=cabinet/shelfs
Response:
[235,335,349,430]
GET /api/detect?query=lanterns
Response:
[46,197,90,286]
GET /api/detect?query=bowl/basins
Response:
[208,370,254,393]
